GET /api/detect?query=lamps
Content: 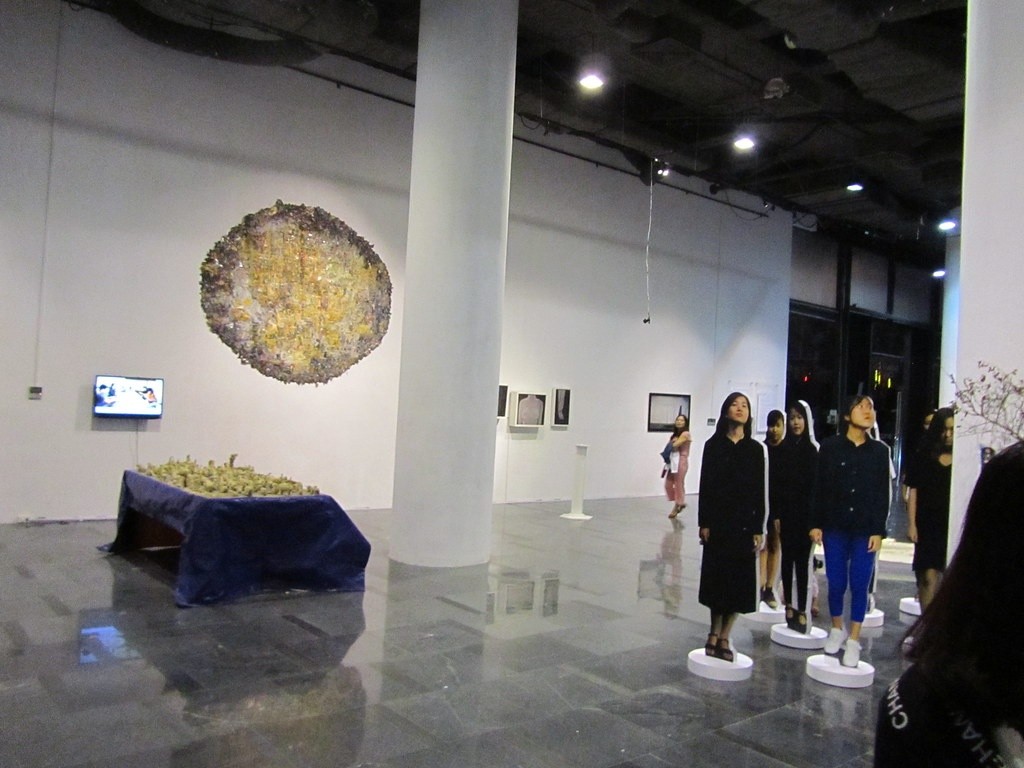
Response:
[639,157,668,186]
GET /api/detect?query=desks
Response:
[95,468,372,609]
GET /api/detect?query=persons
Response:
[661,413,691,519]
[874,439,1024,768]
[698,392,954,668]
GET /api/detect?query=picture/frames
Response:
[509,392,547,428]
[648,393,691,432]
[550,387,571,427]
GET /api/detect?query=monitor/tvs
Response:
[93,376,163,419]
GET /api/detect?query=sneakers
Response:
[842,639,862,666]
[824,623,848,653]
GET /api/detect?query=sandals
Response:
[704,633,734,662]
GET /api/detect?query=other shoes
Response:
[668,509,679,518]
[761,587,777,608]
[677,505,685,513]
[785,607,807,633]
